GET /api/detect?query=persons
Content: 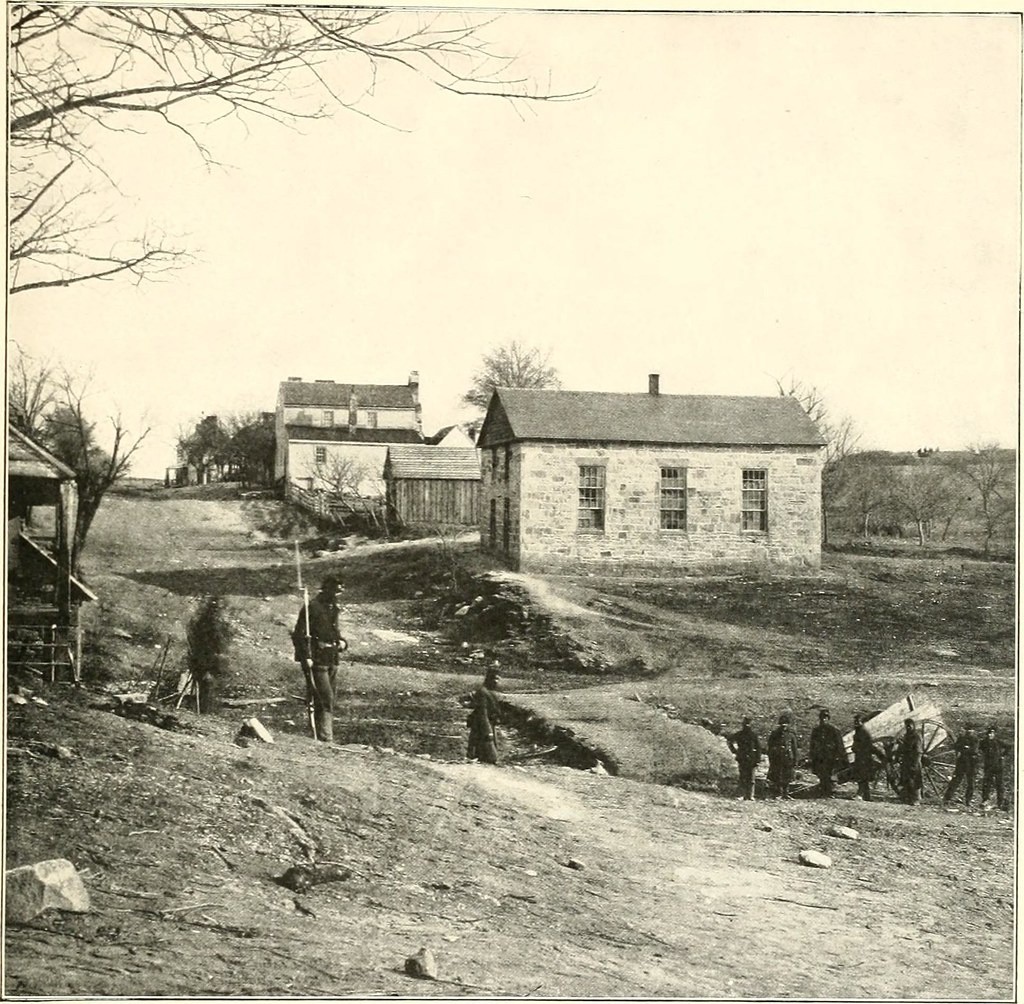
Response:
[467,669,501,765]
[727,709,1012,811]
[293,577,348,742]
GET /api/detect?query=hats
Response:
[778,716,790,723]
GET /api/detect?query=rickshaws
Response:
[791,689,958,800]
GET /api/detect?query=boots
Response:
[907,789,922,805]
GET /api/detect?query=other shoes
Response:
[998,804,1008,811]
[966,800,971,806]
[944,801,949,806]
[852,790,870,800]
[980,800,992,807]
